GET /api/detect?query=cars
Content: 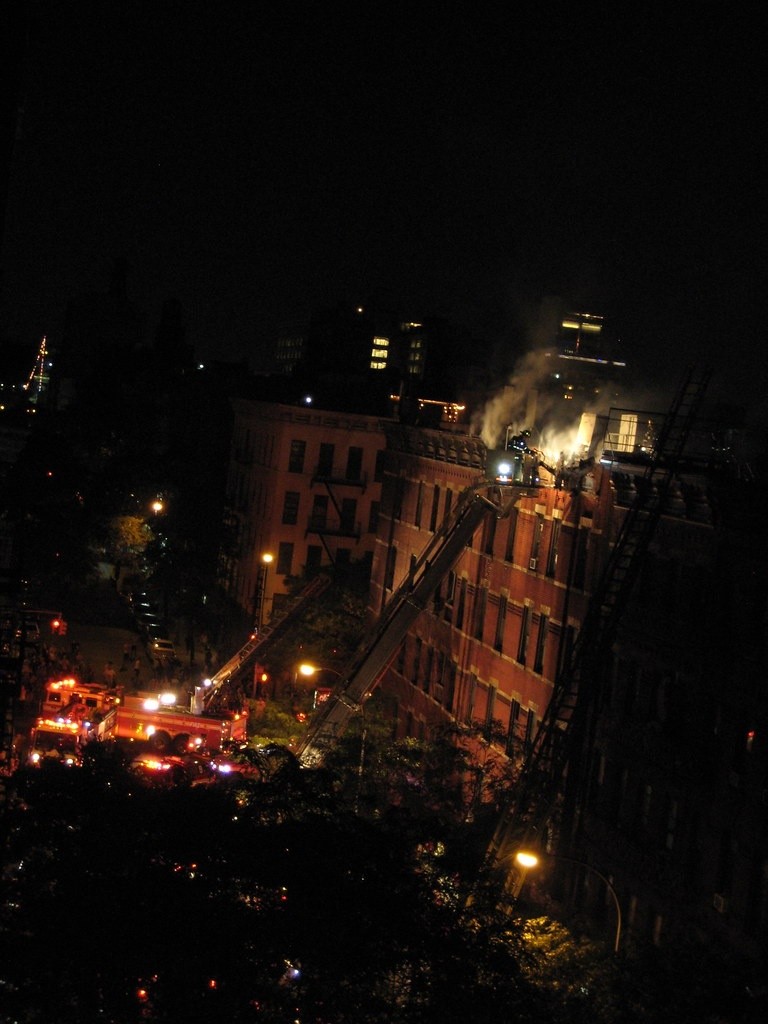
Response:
[116,575,178,668]
[0,603,63,647]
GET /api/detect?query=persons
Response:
[507,429,536,481]
[0,620,270,791]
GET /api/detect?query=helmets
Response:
[520,430,531,438]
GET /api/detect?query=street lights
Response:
[513,851,623,956]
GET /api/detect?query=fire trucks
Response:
[26,455,545,806]
[40,559,350,752]
